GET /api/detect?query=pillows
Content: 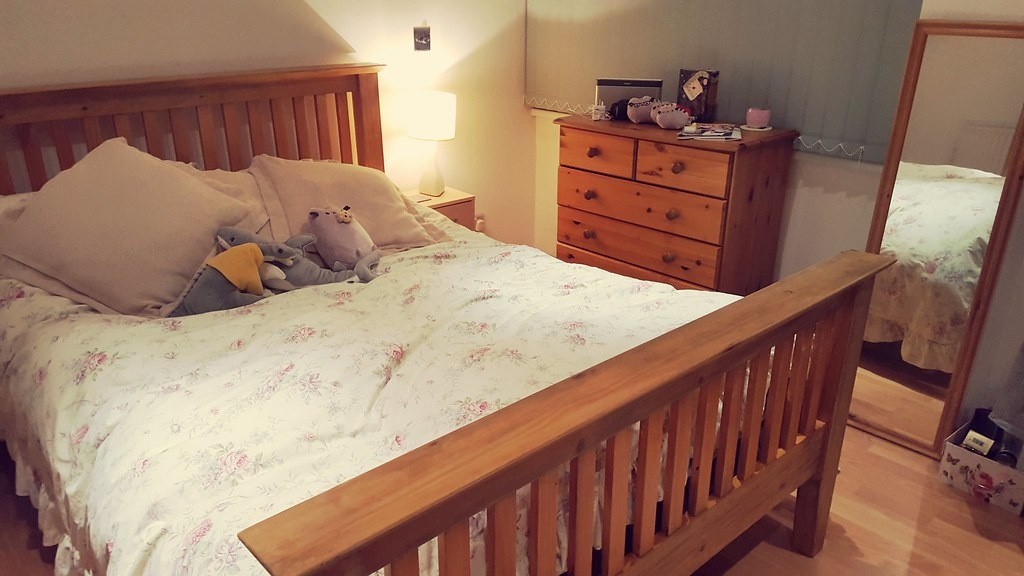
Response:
[0,138,452,319]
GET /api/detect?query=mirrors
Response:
[846,22,1024,460]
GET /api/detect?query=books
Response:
[677,122,741,141]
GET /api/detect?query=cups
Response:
[746,107,770,129]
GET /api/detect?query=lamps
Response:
[407,88,458,197]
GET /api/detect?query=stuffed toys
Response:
[163,242,272,319]
[308,205,383,282]
[217,225,354,291]
[627,96,691,129]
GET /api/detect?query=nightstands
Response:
[402,186,476,232]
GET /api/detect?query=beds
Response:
[863,161,1006,373]
[0,63,897,576]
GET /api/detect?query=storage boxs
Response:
[937,420,1024,517]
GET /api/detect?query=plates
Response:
[740,124,773,131]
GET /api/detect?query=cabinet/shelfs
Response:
[552,113,799,296]
